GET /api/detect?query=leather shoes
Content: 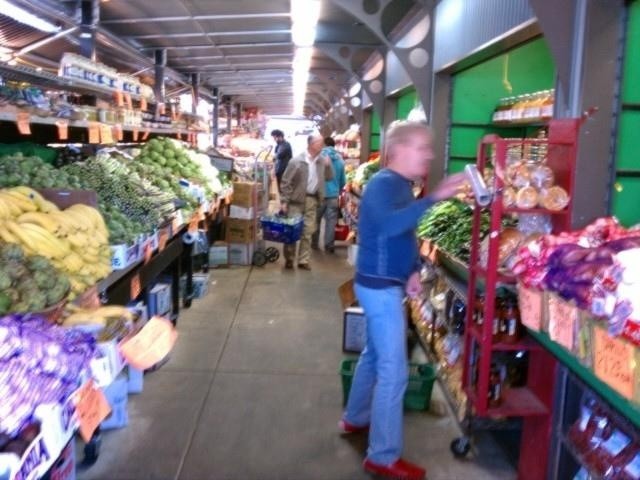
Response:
[286,261,311,270]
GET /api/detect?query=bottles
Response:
[535,130,545,139]
[492,89,556,122]
[472,293,523,343]
[474,349,529,409]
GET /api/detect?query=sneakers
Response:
[363,455,426,480]
[337,419,371,434]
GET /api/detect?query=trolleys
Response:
[451,106,597,478]
[252,144,279,267]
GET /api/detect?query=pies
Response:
[501,159,570,212]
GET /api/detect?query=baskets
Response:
[261,214,304,243]
[340,359,436,411]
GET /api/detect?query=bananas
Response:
[0,185,114,295]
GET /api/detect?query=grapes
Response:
[0,151,159,245]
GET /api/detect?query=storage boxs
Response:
[506,274,640,413]
[75,196,232,331]
[203,179,273,270]
[333,271,409,358]
[75,321,145,430]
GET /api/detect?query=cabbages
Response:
[134,135,196,190]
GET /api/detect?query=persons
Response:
[336,121,469,480]
[271,130,292,192]
[279,134,334,271]
[311,137,346,253]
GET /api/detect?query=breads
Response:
[497,227,543,268]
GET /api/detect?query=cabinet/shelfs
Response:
[512,309,639,480]
[402,226,534,459]
[1,59,208,144]
[442,115,584,480]
